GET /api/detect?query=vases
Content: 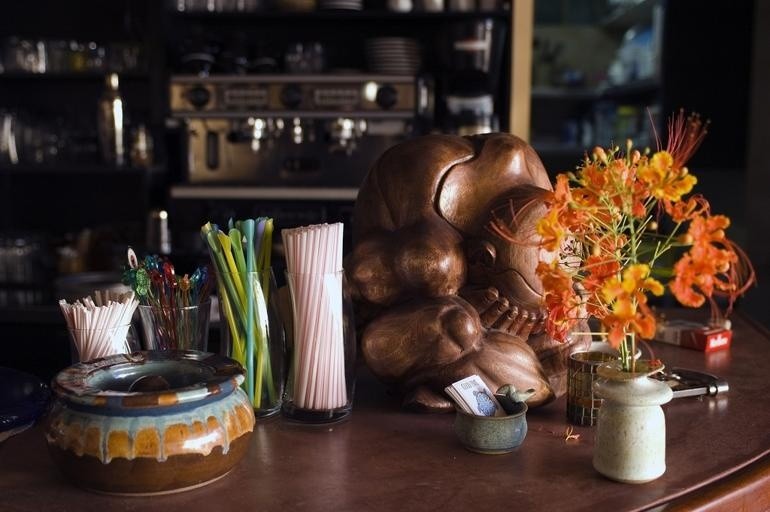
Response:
[592,360,672,487]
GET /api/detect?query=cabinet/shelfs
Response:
[166,72,419,202]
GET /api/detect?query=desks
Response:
[0,294,770,512]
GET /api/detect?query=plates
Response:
[368,34,424,75]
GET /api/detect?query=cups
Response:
[454,393,530,459]
[137,298,211,353]
[66,322,142,366]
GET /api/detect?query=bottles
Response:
[213,266,288,422]
[282,268,357,425]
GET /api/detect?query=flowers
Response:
[486,105,757,372]
